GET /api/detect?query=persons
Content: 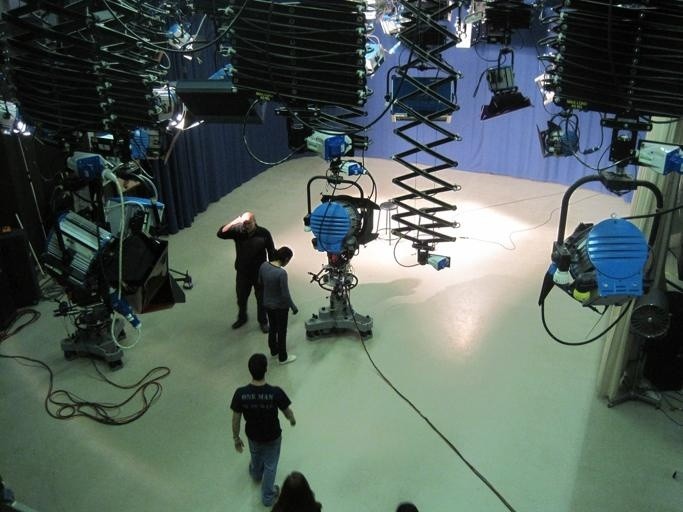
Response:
[256,246,299,365]
[216,210,276,334]
[268,470,324,512]
[230,353,297,508]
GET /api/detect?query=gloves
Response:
[291,304,298,315]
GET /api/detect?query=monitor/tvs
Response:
[176,81,266,125]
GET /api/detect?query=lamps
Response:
[479,47,532,120]
[539,173,662,345]
[301,171,381,257]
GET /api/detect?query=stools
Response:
[375,201,401,247]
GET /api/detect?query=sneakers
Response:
[249,463,262,485]
[260,322,269,334]
[271,353,279,359]
[264,485,280,506]
[232,318,248,330]
[279,355,296,365]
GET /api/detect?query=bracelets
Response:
[232,434,241,441]
